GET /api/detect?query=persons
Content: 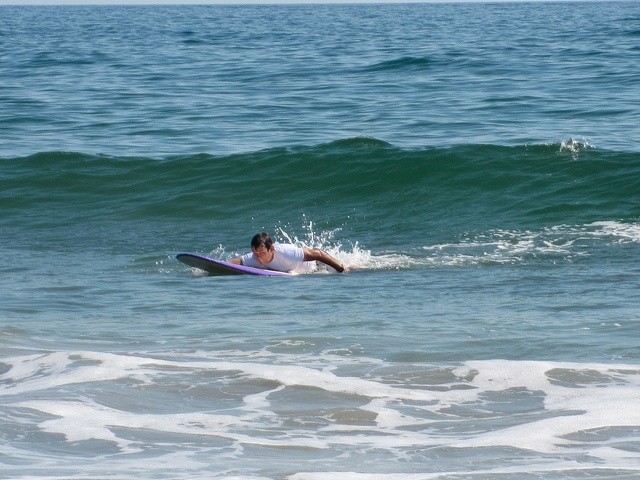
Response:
[224,231,351,277]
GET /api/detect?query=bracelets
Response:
[338,264,345,272]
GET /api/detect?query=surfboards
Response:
[175,252,291,277]
